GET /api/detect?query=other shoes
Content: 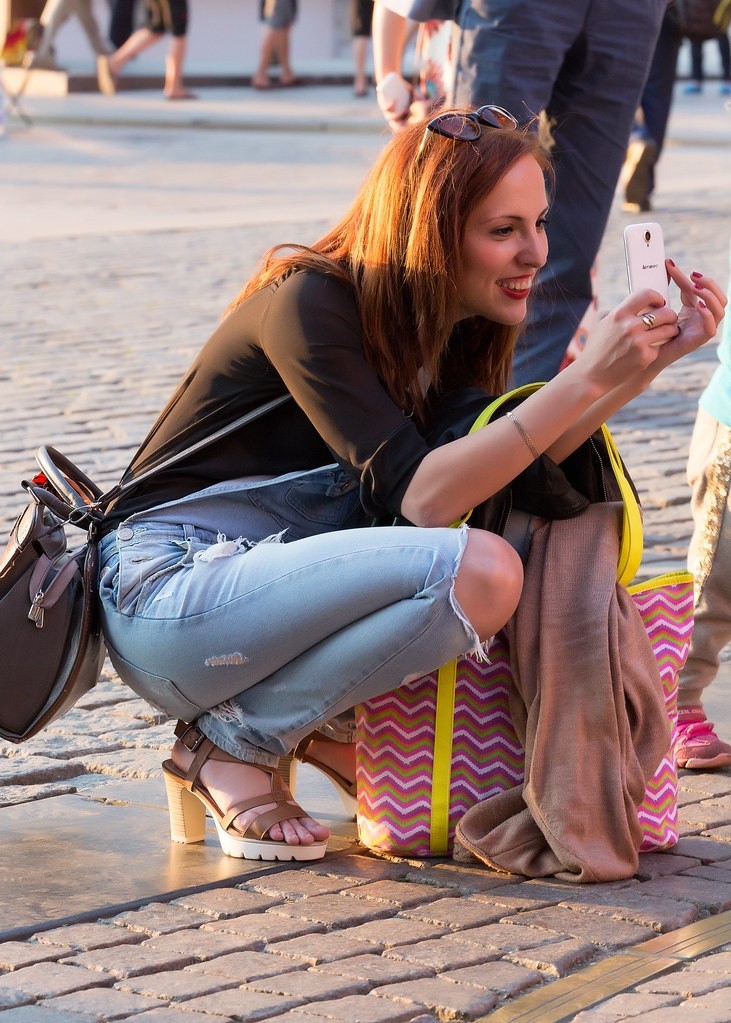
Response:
[684,85,702,93]
[721,85,730,94]
[96,54,116,94]
[622,192,650,213]
[279,78,302,87]
[164,88,195,99]
[253,80,276,90]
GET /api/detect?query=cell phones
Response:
[623,222,673,346]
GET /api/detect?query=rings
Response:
[640,312,656,328]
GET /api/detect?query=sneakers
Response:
[675,706,731,768]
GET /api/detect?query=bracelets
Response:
[505,412,545,464]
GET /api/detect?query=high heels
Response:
[278,729,356,819]
[162,719,327,860]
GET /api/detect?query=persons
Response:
[101,107,727,862]
[667,247,731,770]
[0,0,731,386]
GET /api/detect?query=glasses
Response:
[413,104,518,163]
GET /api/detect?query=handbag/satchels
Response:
[0,445,102,744]
[355,381,695,857]
